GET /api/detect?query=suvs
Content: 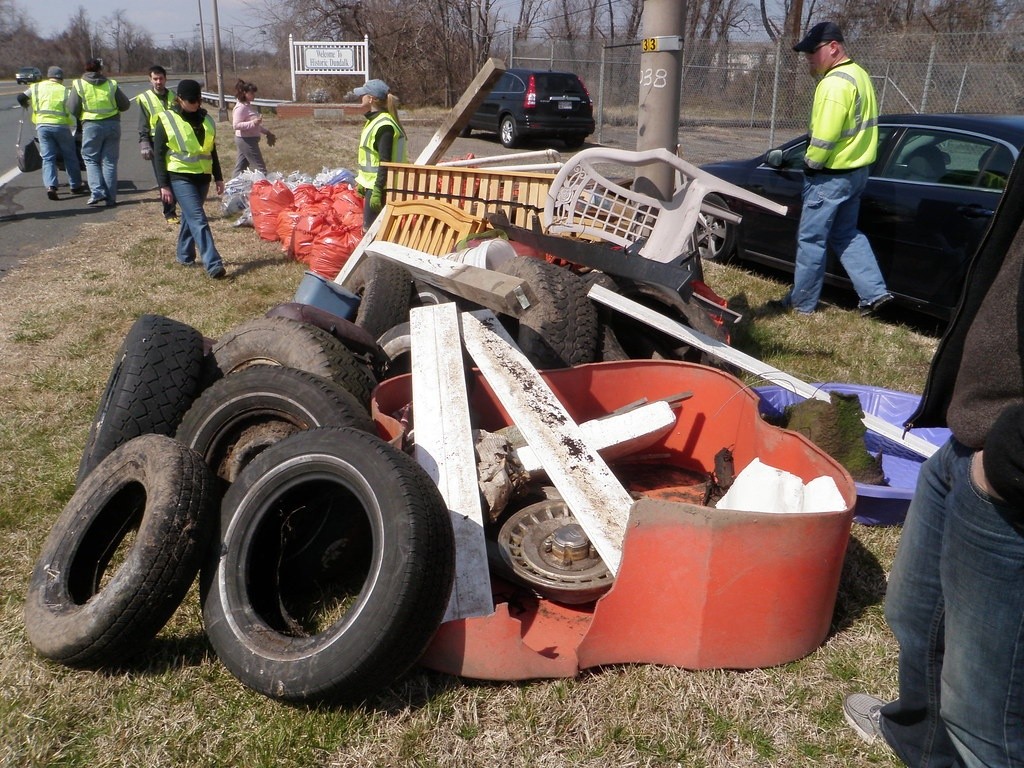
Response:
[457,68,596,149]
[16,67,42,84]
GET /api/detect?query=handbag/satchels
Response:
[16,138,43,173]
[220,165,366,280]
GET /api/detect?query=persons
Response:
[154,79,225,279]
[66,57,130,204]
[17,66,85,199]
[768,20,897,320]
[841,146,1024,768]
[232,78,272,179]
[353,79,409,235]
[136,65,180,223]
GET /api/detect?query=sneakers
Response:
[841,693,895,755]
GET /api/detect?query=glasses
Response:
[804,41,831,54]
[187,97,204,105]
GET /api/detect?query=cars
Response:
[694,113,1024,325]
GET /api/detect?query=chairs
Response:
[971,144,1015,186]
[905,144,947,182]
[543,147,788,325]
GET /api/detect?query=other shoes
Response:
[47,187,58,200]
[764,298,787,311]
[210,268,226,279]
[70,188,80,194]
[166,217,180,224]
[175,256,195,266]
[106,201,116,208]
[87,197,107,205]
[854,294,893,317]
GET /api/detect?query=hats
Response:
[177,80,201,99]
[792,22,844,52]
[48,66,63,80]
[354,78,390,100]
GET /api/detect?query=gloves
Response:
[140,137,154,161]
[370,187,383,213]
